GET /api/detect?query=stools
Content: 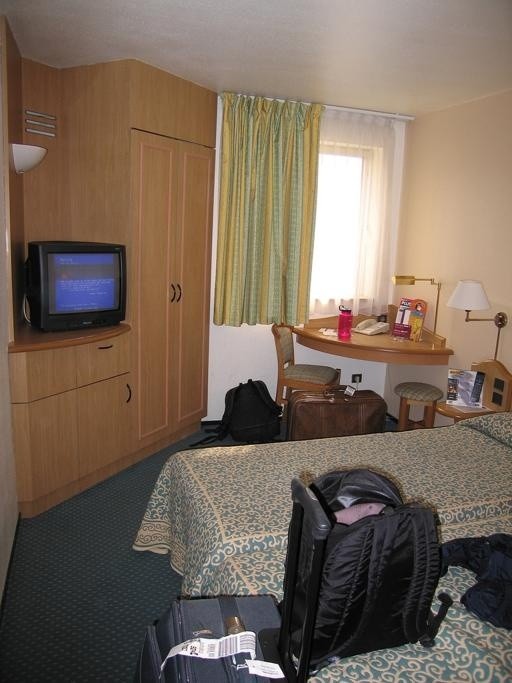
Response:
[394,382,442,434]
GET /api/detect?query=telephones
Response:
[351,318,390,336]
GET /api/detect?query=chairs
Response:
[272,323,336,420]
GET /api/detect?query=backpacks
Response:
[189,379,283,447]
[278,468,454,669]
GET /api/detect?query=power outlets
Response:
[352,374,361,383]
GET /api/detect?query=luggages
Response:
[285,382,388,442]
[134,475,331,682]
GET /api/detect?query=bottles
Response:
[338,305,352,342]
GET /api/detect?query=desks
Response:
[290,303,454,365]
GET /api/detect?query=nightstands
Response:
[438,358,512,423]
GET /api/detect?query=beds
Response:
[210,516,510,682]
[173,414,511,594]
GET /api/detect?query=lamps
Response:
[394,274,441,340]
[448,280,508,329]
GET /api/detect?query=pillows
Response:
[458,413,512,448]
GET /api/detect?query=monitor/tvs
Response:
[23,241,127,332]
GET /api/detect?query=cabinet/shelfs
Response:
[3,12,219,521]
